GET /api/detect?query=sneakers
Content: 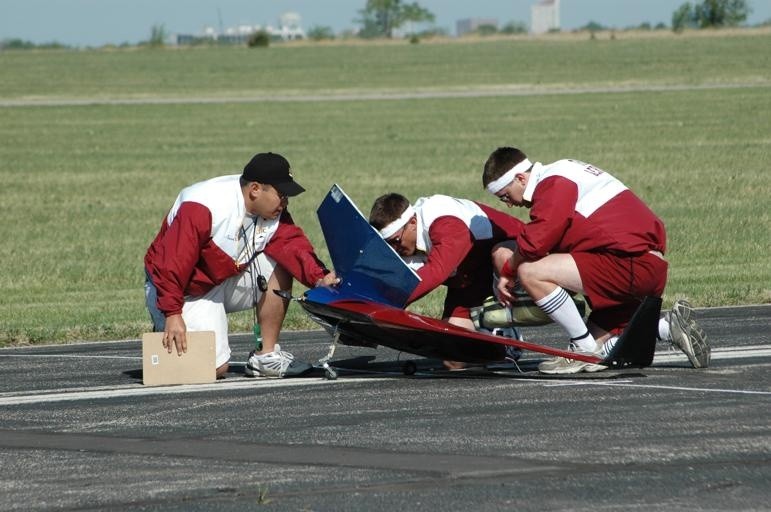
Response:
[667,299,711,368]
[538,343,609,375]
[245,344,313,379]
[492,327,524,361]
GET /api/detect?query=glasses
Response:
[385,226,405,245]
[499,193,511,202]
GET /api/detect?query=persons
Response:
[369,193,585,370]
[143,151,341,377]
[482,147,711,374]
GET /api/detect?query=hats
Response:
[242,152,306,196]
